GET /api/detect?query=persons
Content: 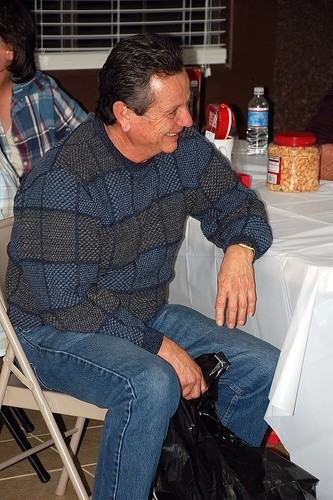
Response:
[305,87,333,181]
[0,0,95,388]
[5,31,281,500]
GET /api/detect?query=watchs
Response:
[228,242,256,257]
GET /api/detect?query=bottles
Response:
[267,129,319,193]
[205,102,238,140]
[246,86,269,156]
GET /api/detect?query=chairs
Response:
[0,216,108,500]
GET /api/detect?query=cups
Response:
[232,153,268,188]
[208,136,234,163]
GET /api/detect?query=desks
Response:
[168,140,333,500]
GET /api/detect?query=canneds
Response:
[265,131,320,193]
